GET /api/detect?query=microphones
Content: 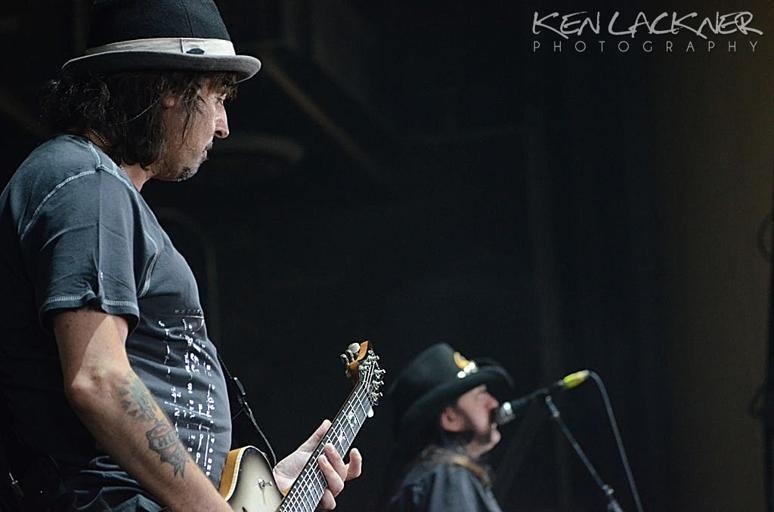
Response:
[495,370,587,425]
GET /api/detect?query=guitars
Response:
[217,341,386,511]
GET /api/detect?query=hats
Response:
[61,0,262,84]
[387,343,495,445]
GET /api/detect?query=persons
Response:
[1,0,365,512]
[381,340,516,512]
